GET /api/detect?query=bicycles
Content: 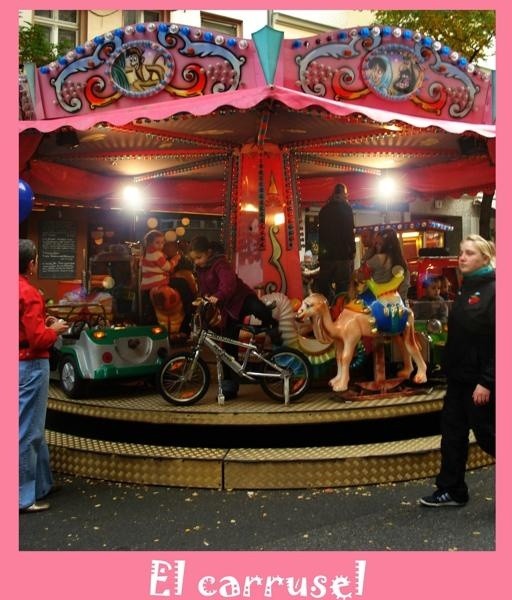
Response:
[156,296,313,406]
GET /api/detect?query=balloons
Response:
[19,180,33,223]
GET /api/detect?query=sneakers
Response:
[217,392,236,399]
[419,491,462,506]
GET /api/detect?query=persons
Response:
[19,239,69,514]
[300,251,320,295]
[361,227,378,259]
[436,276,457,300]
[139,230,188,327]
[189,236,282,401]
[413,278,449,326]
[419,235,495,506]
[318,183,354,301]
[365,229,410,302]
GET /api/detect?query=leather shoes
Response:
[24,501,49,512]
